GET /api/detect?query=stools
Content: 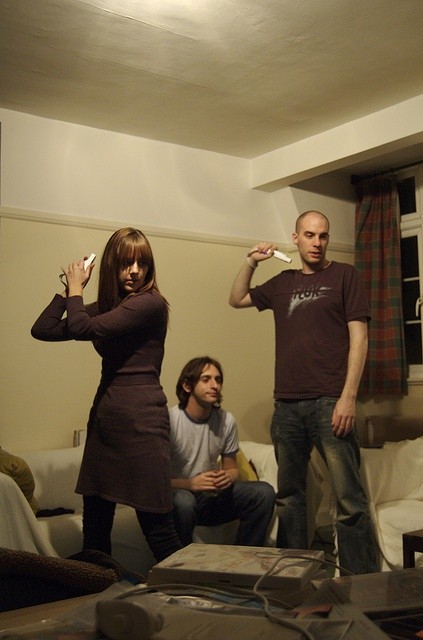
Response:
[402,532,421,574]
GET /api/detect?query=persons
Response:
[167,356,276,547]
[31,227,183,565]
[229,210,381,576]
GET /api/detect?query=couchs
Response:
[0,437,289,556]
[330,415,422,578]
[1,548,131,612]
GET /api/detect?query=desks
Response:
[1,569,423,640]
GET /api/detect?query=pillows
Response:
[0,450,36,508]
